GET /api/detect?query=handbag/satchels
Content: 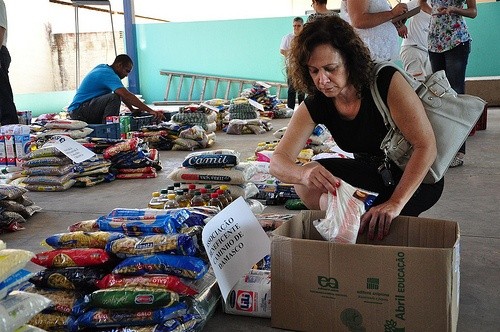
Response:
[368,60,488,185]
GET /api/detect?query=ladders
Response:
[153,71,300,105]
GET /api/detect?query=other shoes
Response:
[449,156,463,168]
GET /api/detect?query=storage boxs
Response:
[270,210,460,331]
[86,122,119,140]
[127,114,155,131]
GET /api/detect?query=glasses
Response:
[311,2,315,7]
[293,25,302,27]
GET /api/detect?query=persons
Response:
[417,0,478,168]
[68,55,166,124]
[306,0,340,23]
[269,16,445,241]
[280,17,306,110]
[396,0,433,84]
[340,0,408,64]
[0,0,19,126]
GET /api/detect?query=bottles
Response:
[205,185,233,211]
[149,186,179,208]
[27,137,45,151]
[175,181,210,207]
